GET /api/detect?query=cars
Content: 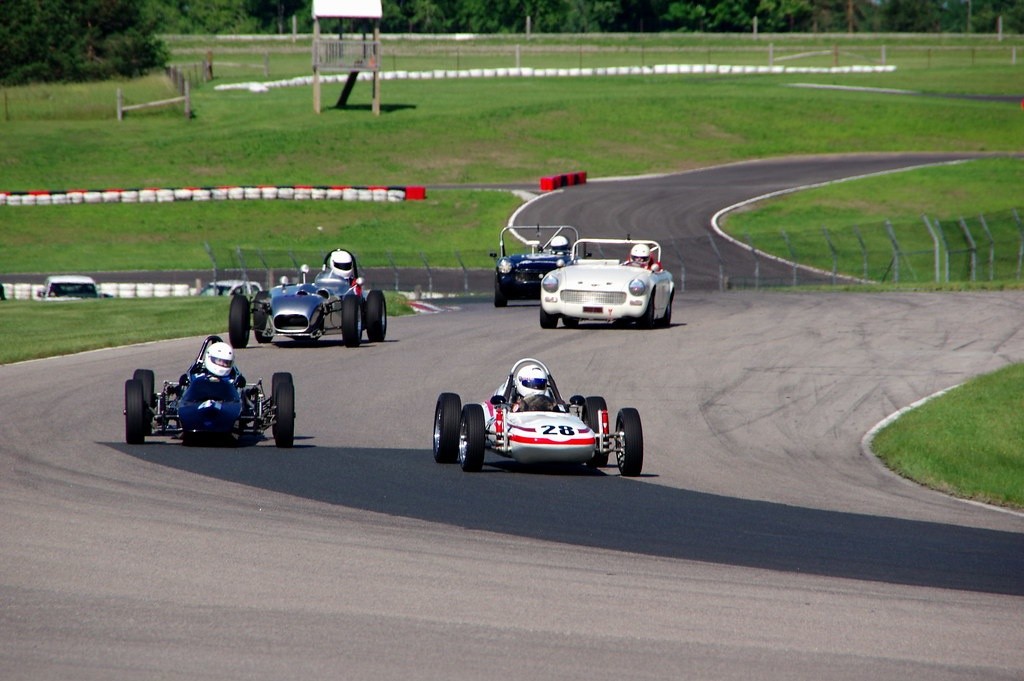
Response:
[37,275,101,301]
[540,237,675,328]
[198,279,262,296]
[492,223,580,309]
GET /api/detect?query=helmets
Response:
[629,244,650,265]
[329,251,353,280]
[515,365,548,401]
[549,236,568,253]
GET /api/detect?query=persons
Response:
[627,242,662,271]
[191,341,239,396]
[329,250,362,296]
[548,234,570,254]
[511,364,556,411]
